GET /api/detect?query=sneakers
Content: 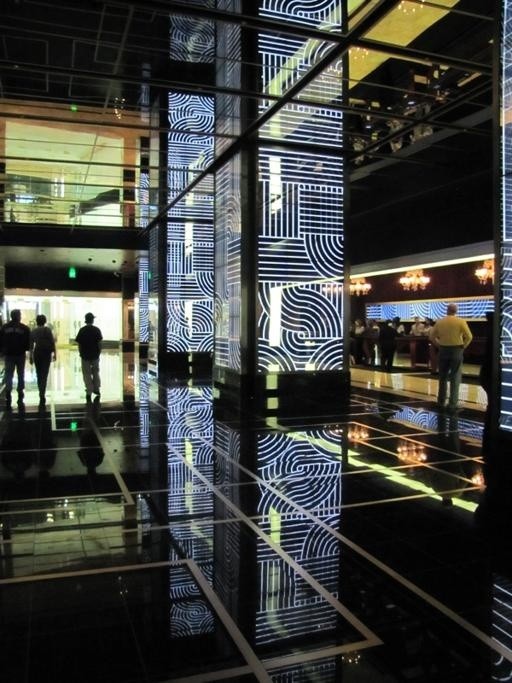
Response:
[437,400,464,409]
[85,387,102,399]
[4,393,48,406]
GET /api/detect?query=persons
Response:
[430,405,470,505]
[1,408,33,483]
[29,314,56,409]
[76,399,105,476]
[0,308,32,408]
[349,315,437,375]
[73,313,102,398]
[427,302,474,405]
[29,407,60,478]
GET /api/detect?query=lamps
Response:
[321,257,495,296]
[348,424,485,486]
